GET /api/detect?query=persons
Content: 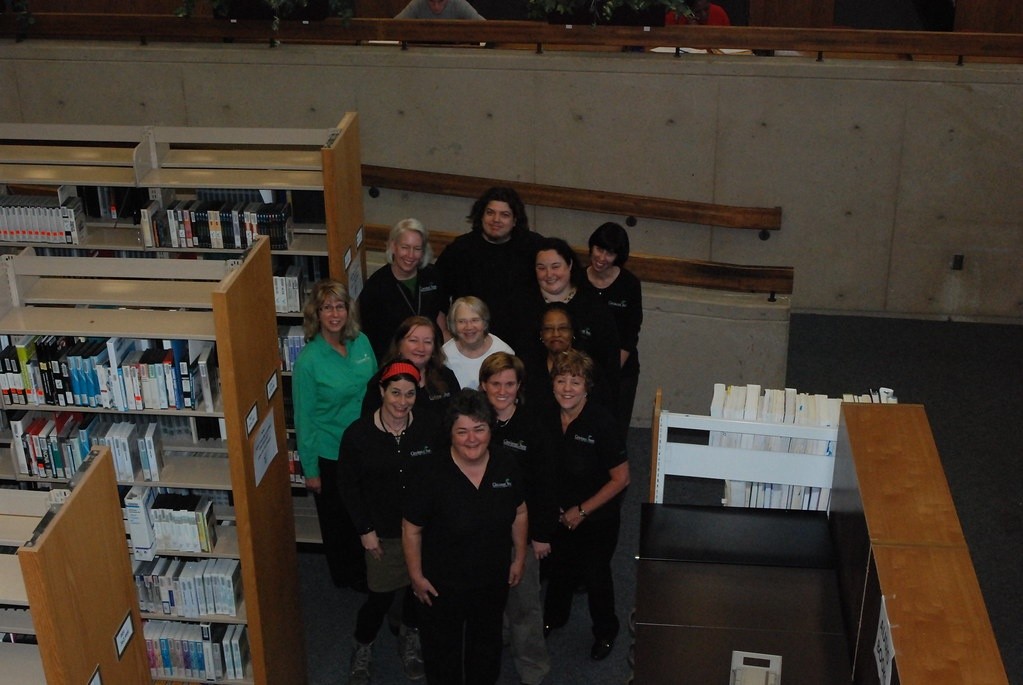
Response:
[394,0,485,20]
[291,186,644,685]
[665,0,729,26]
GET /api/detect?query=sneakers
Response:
[348,637,374,685]
[397,624,424,680]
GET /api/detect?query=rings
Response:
[569,526,571,528]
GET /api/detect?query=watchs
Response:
[578,505,587,517]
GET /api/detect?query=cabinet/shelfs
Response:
[629,386,1008,685]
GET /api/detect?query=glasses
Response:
[318,305,346,312]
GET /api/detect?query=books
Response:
[287,439,306,483]
[140,199,292,251]
[0,194,88,246]
[277,325,305,371]
[0,335,248,681]
[708,384,881,511]
[273,265,305,313]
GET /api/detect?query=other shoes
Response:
[543,603,571,629]
[591,635,613,659]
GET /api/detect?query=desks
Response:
[0,111,365,685]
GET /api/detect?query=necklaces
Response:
[383,418,405,440]
